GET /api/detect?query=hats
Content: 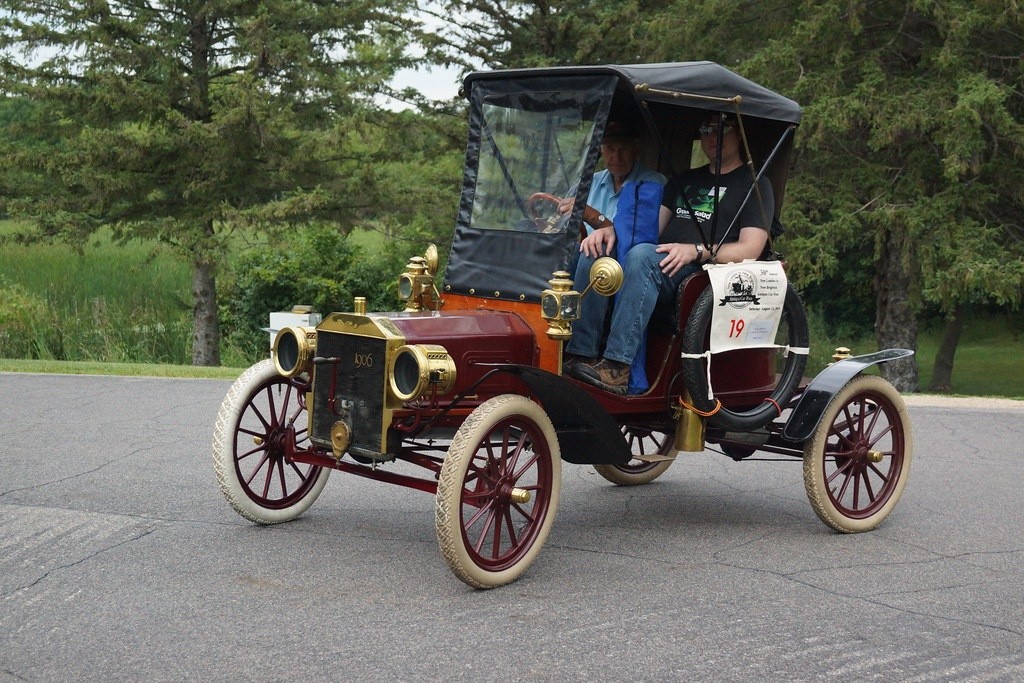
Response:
[599,116,640,145]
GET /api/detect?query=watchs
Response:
[694,243,702,264]
[591,214,605,229]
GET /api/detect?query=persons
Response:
[533,122,668,234]
[562,109,775,395]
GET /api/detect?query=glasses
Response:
[697,121,738,136]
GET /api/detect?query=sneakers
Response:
[563,353,631,394]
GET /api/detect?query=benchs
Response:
[644,259,780,334]
